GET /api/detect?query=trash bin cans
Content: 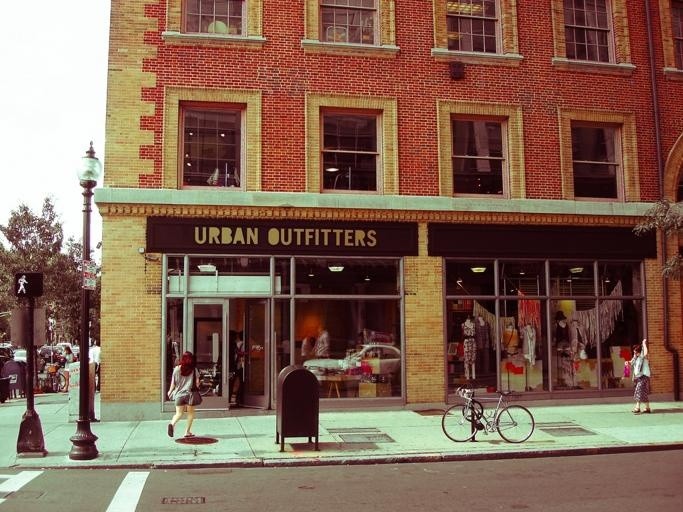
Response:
[275,364,320,451]
[1,360,25,400]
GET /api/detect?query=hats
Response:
[554,310,567,321]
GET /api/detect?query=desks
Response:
[317,374,361,398]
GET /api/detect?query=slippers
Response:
[168,424,174,437]
[185,432,195,437]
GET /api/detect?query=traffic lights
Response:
[15,272,45,296]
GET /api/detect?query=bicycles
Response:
[440,385,538,444]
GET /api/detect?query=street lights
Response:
[71,140,103,461]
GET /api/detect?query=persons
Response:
[298,331,313,363]
[311,320,332,359]
[234,329,253,408]
[218,329,239,402]
[164,349,202,439]
[459,313,590,385]
[624,338,651,415]
[87,336,100,392]
[59,346,75,393]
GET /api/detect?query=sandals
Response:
[642,408,650,413]
[632,407,640,412]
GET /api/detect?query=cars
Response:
[300,344,402,384]
[0,335,82,400]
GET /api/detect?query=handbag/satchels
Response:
[189,369,202,406]
[636,358,643,373]
